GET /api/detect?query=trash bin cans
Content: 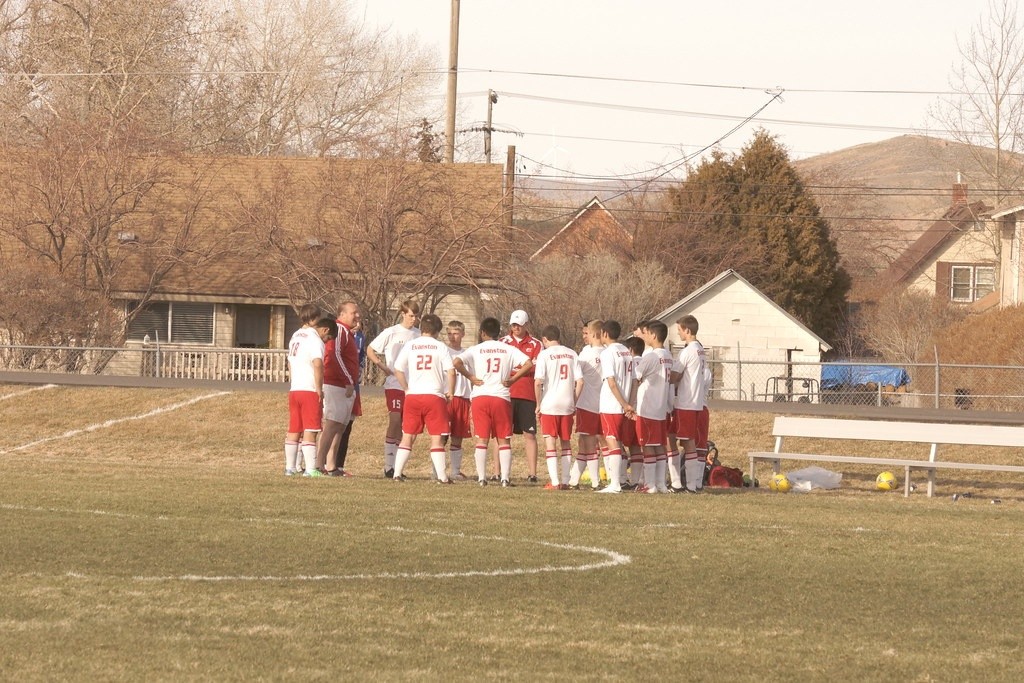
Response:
[821,378,842,403]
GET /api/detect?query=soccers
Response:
[581,470,592,485]
[769,473,791,493]
[598,467,607,483]
[875,471,898,491]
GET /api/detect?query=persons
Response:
[285,301,364,476]
[367,300,712,494]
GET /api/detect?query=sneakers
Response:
[667,483,698,494]
[636,483,668,494]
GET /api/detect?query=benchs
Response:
[747,417,1024,498]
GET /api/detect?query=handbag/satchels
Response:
[708,466,743,488]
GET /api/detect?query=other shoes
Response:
[392,473,413,483]
[501,478,516,487]
[284,463,352,478]
[620,483,640,492]
[477,480,487,487]
[527,476,538,485]
[486,475,501,484]
[450,473,467,481]
[437,478,456,484]
[383,467,407,479]
[543,483,570,490]
[568,484,580,491]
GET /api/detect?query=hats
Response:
[510,310,528,326]
